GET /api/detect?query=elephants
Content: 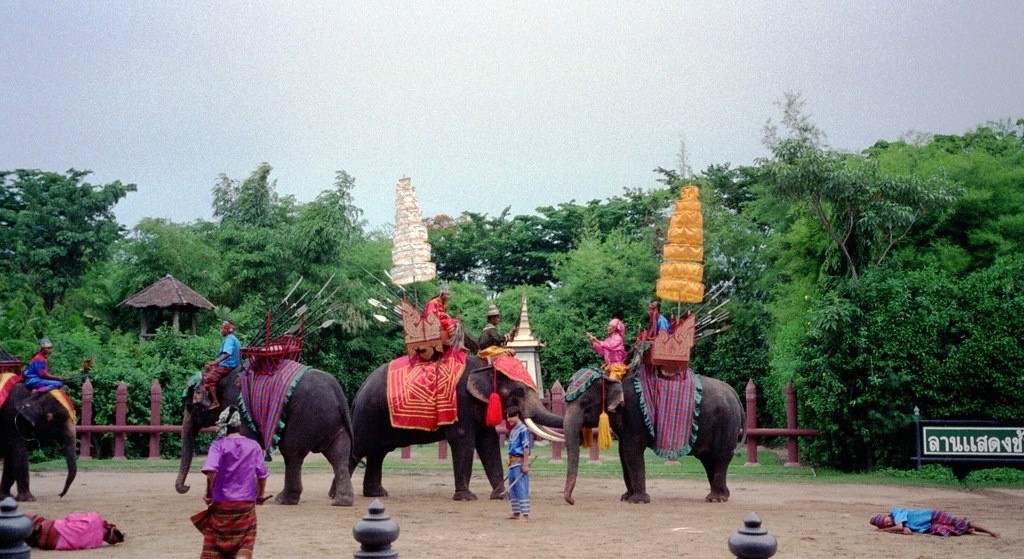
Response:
[1,372,78,503]
[562,363,748,506]
[174,360,366,505]
[327,350,565,501]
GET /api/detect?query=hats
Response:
[439,280,450,294]
[870,515,886,530]
[40,338,52,349]
[487,303,501,316]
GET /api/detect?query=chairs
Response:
[400,296,466,367]
[634,313,695,378]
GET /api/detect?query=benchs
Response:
[239,308,305,373]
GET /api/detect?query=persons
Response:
[870,507,1001,539]
[587,318,627,410]
[504,406,531,521]
[203,320,241,410]
[649,301,671,336]
[190,406,270,559]
[23,338,67,396]
[424,288,470,351]
[479,305,517,357]
[17,513,125,551]
